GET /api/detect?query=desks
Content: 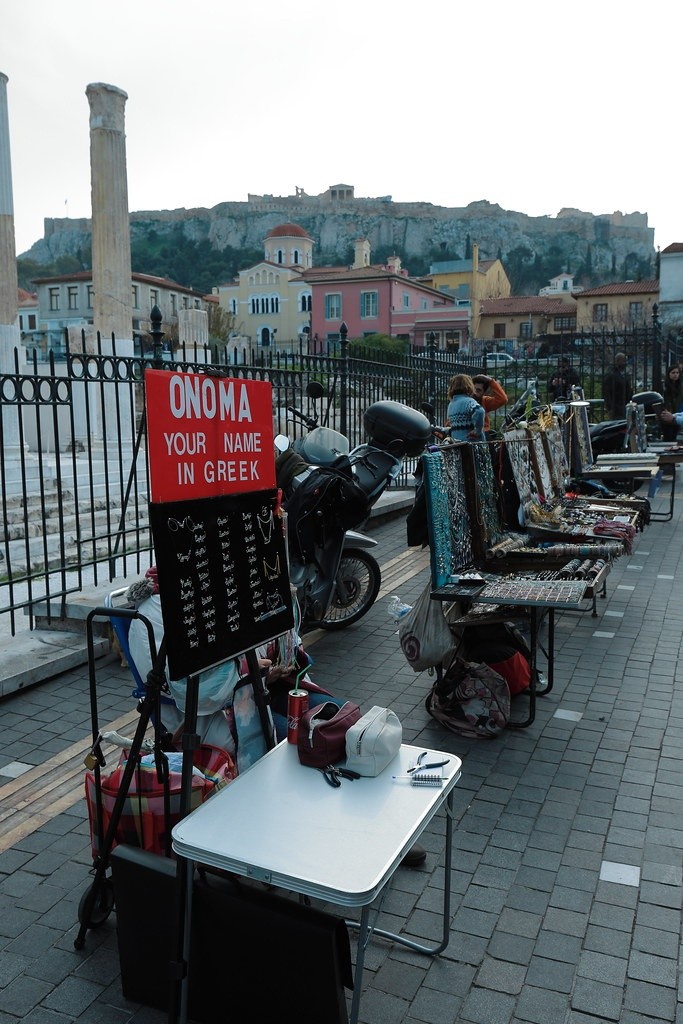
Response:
[172,720,463,1024]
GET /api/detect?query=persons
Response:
[656,364,683,484]
[437,373,510,439]
[659,407,683,426]
[482,339,495,355]
[519,342,534,359]
[537,341,549,360]
[547,357,583,402]
[602,350,634,424]
[445,373,486,444]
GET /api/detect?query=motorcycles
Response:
[506,380,666,491]
[260,381,433,639]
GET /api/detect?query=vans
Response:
[480,353,515,369]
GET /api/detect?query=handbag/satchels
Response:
[346,705,403,777]
[85,743,237,872]
[298,700,361,769]
[396,573,537,739]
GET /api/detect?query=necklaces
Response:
[426,407,654,611]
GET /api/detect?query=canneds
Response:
[287,689,309,745]
